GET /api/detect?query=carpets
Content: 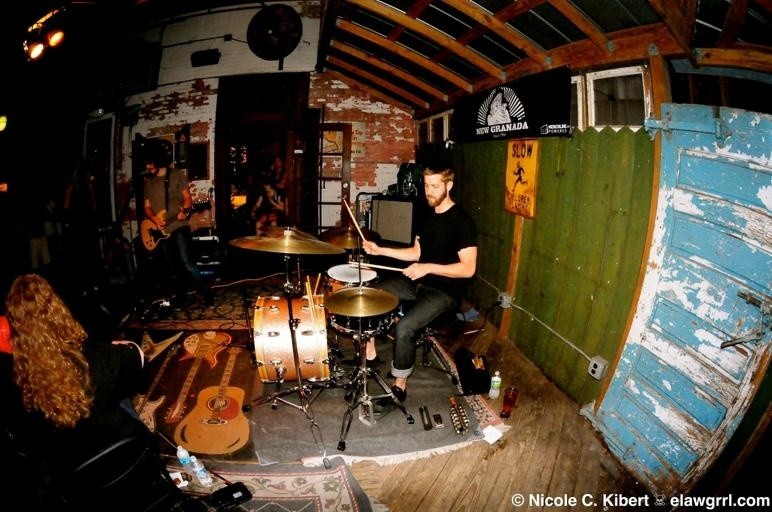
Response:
[161,454,374,512]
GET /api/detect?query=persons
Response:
[252,182,285,234]
[141,150,193,282]
[362,161,475,411]
[6,273,104,433]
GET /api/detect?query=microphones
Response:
[131,169,149,179]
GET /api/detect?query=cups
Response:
[503,387,519,414]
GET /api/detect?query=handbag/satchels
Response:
[452,346,491,394]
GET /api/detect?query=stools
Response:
[401,299,458,386]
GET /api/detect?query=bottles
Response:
[177,447,213,487]
[488,371,502,400]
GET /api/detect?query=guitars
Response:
[131,331,250,455]
[140,201,211,251]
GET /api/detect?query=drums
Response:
[330,287,400,336]
[325,264,377,296]
[254,295,331,382]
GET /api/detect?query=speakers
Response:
[370,193,419,245]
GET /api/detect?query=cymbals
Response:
[324,289,399,318]
[229,226,381,254]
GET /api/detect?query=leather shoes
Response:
[351,356,381,379]
[376,386,406,411]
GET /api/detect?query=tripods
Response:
[244,261,345,426]
[224,300,258,365]
[338,335,414,450]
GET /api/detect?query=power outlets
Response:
[497,292,511,309]
[588,356,608,380]
